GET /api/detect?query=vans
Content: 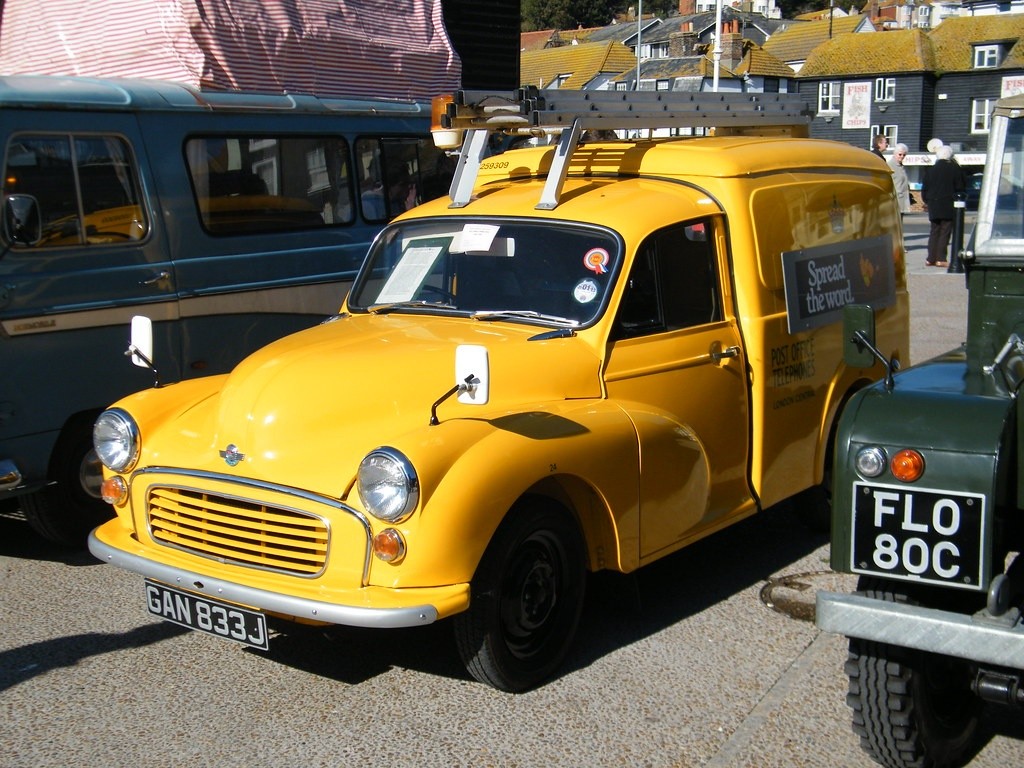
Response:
[0,72,507,550]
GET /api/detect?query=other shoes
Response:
[926,261,933,265]
[936,261,949,267]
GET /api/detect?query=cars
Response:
[758,92,1024,768]
[81,78,916,697]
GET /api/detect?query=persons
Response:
[887,143,912,254]
[367,151,435,218]
[871,134,888,162]
[920,146,964,267]
